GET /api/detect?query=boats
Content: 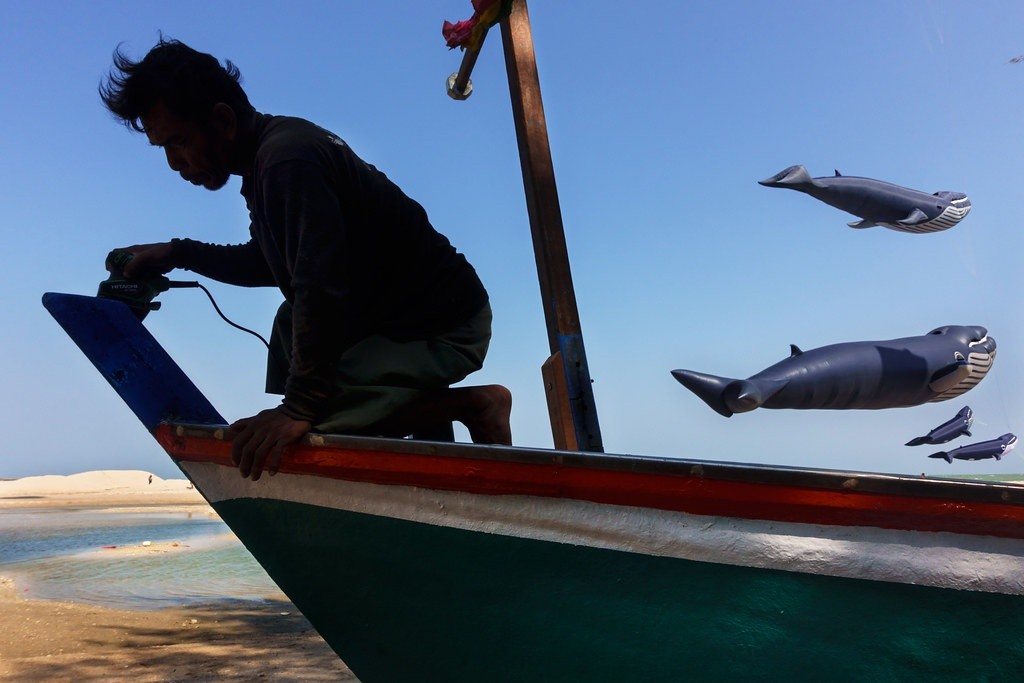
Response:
[43,293,1024,683]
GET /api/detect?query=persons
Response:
[98,40,512,482]
